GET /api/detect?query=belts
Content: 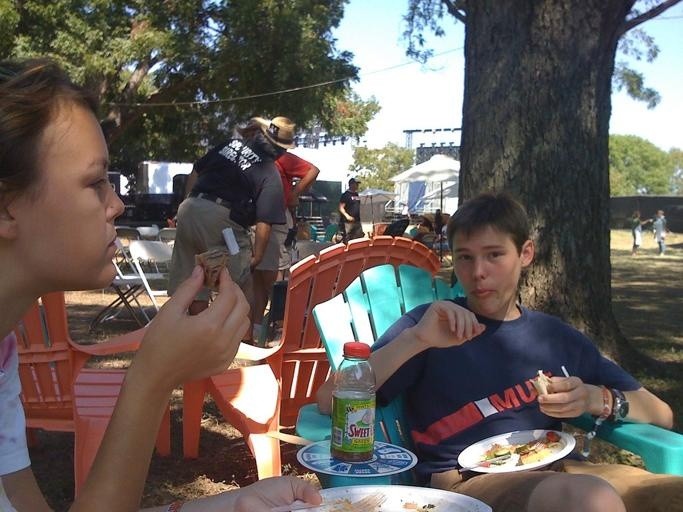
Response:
[188,190,232,209]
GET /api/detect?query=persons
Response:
[0,56,322,512]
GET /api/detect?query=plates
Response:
[288,485,492,511]
[457,428,577,472]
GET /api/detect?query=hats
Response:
[348,178,362,184]
[262,116,298,150]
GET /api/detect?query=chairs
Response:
[181,235,443,480]
[88,210,452,334]
[295,263,683,491]
[13,290,171,502]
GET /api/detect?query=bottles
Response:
[329,341,376,463]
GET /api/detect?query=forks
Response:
[269,489,386,511]
[459,440,544,472]
